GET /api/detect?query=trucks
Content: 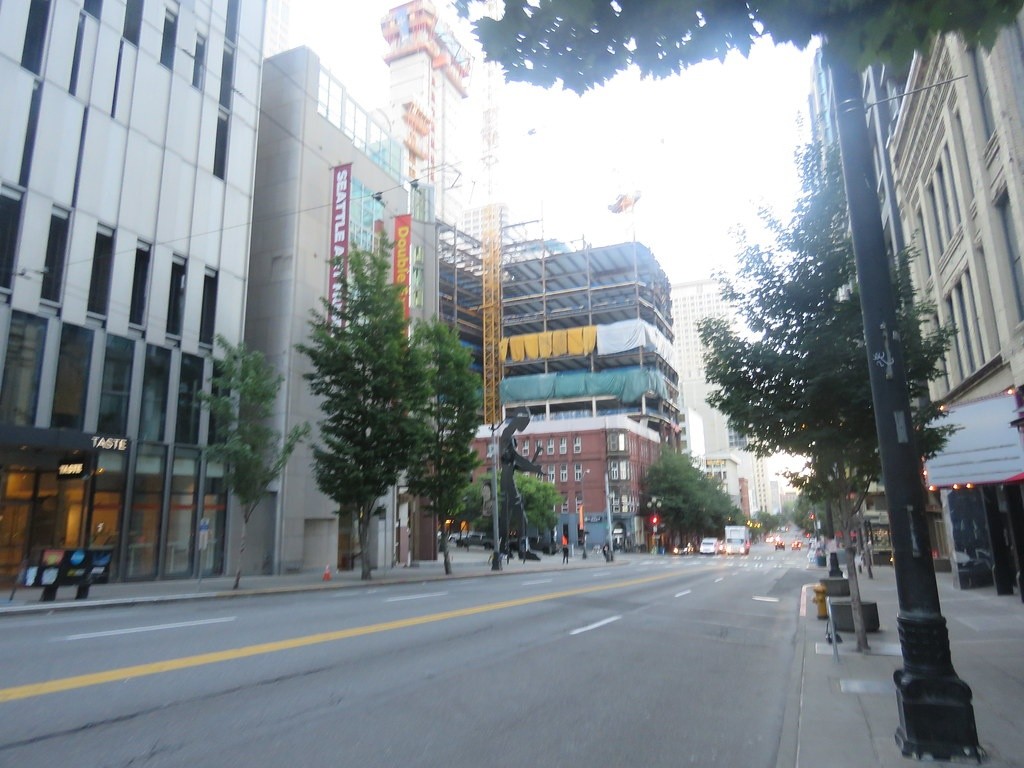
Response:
[724,525,752,556]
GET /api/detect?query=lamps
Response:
[1009,417,1024,433]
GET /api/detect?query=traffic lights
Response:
[810,514,815,520]
[806,532,810,538]
[650,515,659,525]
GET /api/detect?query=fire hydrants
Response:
[811,583,829,621]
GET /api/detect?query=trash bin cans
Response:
[815,555,827,567]
[659,546,667,555]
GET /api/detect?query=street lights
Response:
[647,496,663,555]
[487,413,529,570]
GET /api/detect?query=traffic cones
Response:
[321,562,331,582]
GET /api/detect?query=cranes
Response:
[474,53,644,519]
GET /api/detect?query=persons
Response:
[563,546,571,563]
[602,544,611,562]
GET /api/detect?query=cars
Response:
[673,542,697,555]
[699,537,725,554]
[766,525,803,549]
[438,528,557,556]
